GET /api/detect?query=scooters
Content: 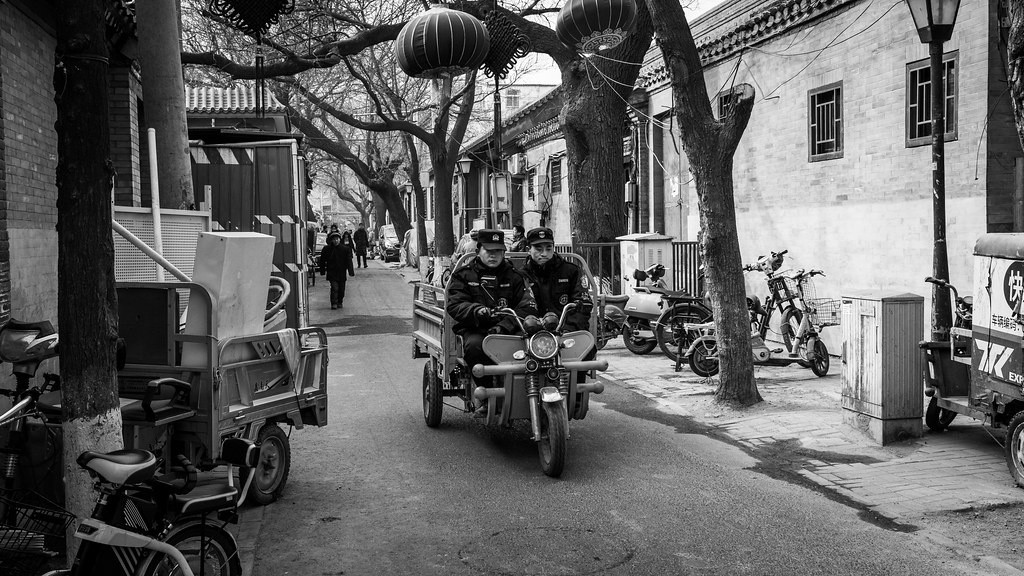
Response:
[620,262,761,355]
[596,262,670,350]
[683,269,841,386]
[648,248,804,373]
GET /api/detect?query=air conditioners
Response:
[507,153,527,175]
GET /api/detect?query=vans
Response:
[378,225,401,263]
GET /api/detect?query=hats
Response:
[478,229,507,251]
[329,233,340,237]
[527,228,554,245]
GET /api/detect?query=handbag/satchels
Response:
[451,253,460,266]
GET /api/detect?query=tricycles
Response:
[306,223,317,286]
[1,281,330,528]
[410,251,608,478]
[917,232,1024,490]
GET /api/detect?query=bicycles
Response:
[1,388,242,576]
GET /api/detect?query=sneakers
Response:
[575,400,589,412]
[474,404,498,418]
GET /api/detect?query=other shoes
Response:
[332,304,343,309]
[364,265,368,268]
[357,265,361,268]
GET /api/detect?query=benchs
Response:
[34,373,195,452]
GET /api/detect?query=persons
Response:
[446,229,539,416]
[354,223,369,268]
[321,224,339,244]
[341,231,356,258]
[462,230,479,263]
[509,225,528,258]
[320,232,355,310]
[518,228,598,409]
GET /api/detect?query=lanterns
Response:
[556,0,637,54]
[394,7,490,102]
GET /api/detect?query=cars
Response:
[307,233,328,268]
